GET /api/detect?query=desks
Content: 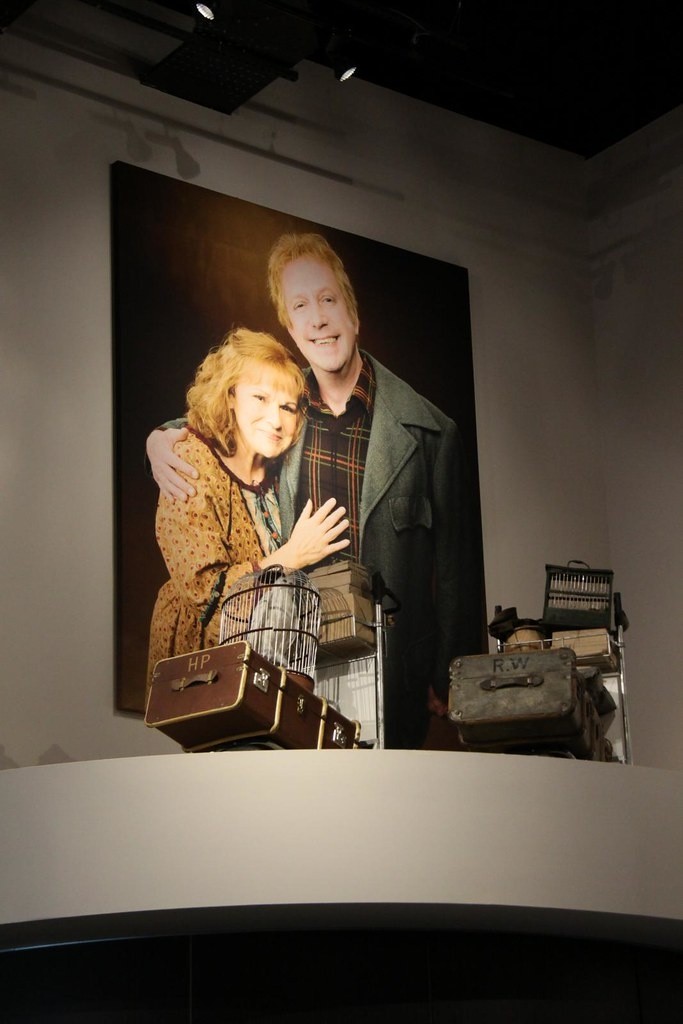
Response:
[0,750,683,1024]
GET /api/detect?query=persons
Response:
[147,230,464,750]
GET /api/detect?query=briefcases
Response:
[448,648,604,760]
[146,641,361,754]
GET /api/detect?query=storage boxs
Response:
[552,630,618,673]
